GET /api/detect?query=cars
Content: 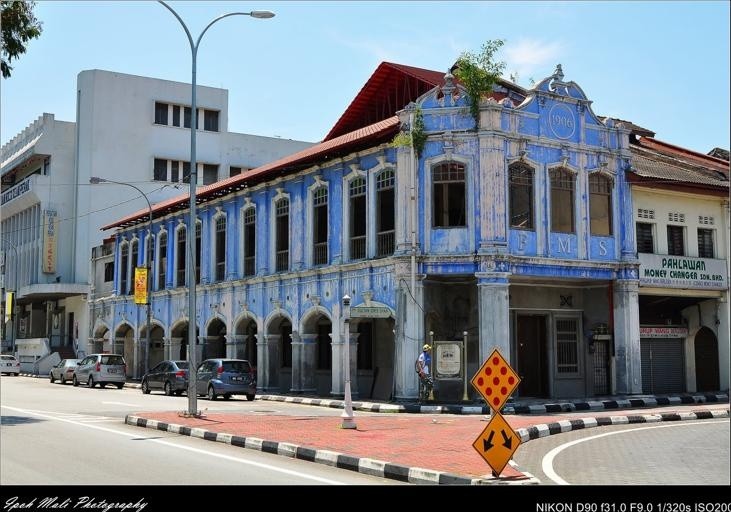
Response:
[49,357,81,384]
[140,360,192,397]
[187,356,258,403]
[0,355,21,376]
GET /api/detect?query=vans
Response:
[74,352,128,389]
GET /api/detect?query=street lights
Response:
[88,177,153,376]
[156,1,276,416]
[339,292,359,431]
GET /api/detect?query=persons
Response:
[415,344,433,405]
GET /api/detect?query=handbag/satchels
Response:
[415,353,426,373]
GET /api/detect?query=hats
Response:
[423,344,431,352]
